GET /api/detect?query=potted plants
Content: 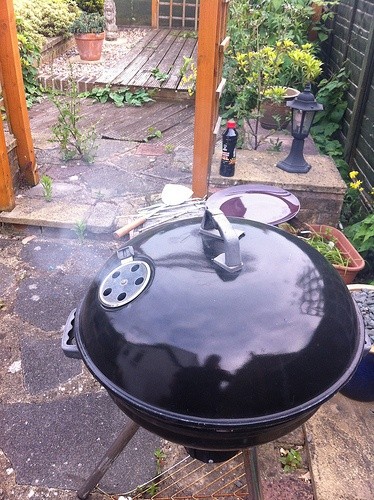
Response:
[69,12,106,61]
[236,40,323,130]
[278,222,365,284]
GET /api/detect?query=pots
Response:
[61,208,365,449]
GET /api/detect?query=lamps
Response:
[276,82,323,173]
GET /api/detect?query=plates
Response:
[204,184,301,226]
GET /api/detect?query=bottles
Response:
[219,121,239,178]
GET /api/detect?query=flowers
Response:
[347,169,365,202]
